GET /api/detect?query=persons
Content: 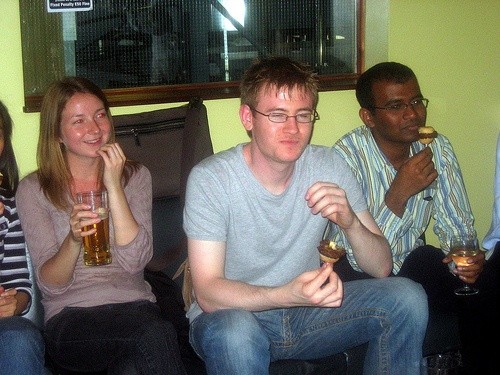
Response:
[481,130,500,280]
[331,62,500,375]
[179,55,429,375]
[0,100,44,375]
[17,75,186,375]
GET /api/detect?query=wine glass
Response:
[450,234,479,296]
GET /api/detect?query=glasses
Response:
[370,98,429,113]
[246,103,319,122]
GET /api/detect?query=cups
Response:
[76,190,112,266]
